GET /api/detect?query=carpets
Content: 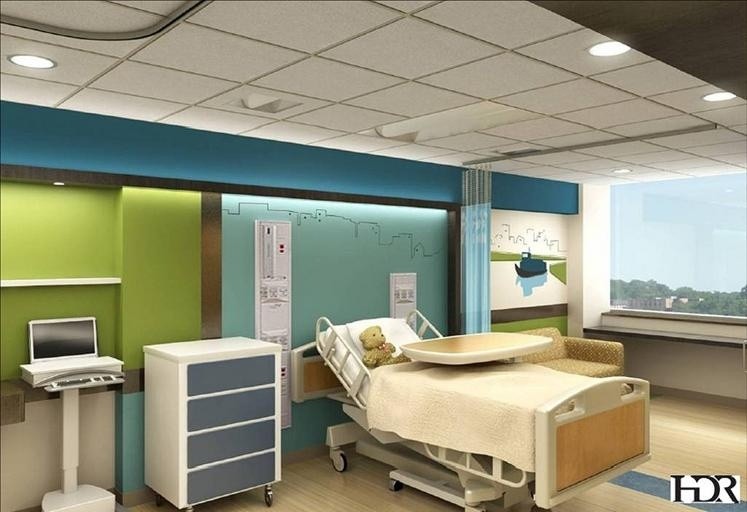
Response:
[607,469,746,511]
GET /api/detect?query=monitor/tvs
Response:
[29,317,99,364]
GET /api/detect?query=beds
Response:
[314,310,651,511]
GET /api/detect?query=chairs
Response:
[513,327,623,378]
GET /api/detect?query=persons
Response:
[359,325,412,369]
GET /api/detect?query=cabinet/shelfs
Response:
[143,338,281,511]
